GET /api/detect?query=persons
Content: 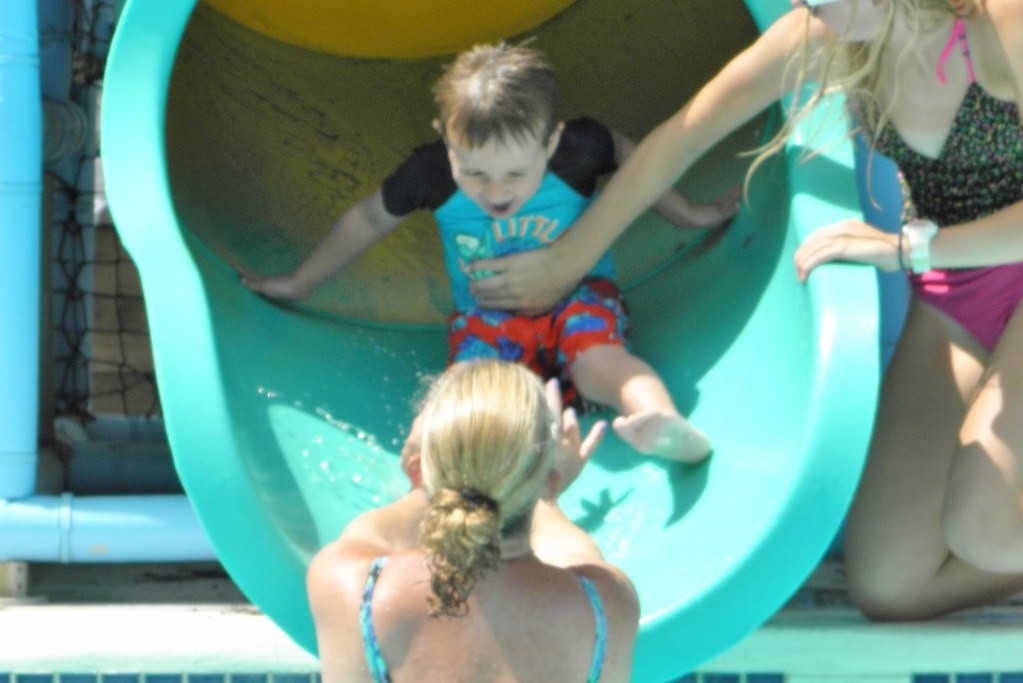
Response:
[461,0,1023,612]
[297,354,642,683]
[231,42,755,486]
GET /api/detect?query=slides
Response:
[97,0,880,683]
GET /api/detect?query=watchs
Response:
[901,218,937,274]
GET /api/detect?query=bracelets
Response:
[894,229,912,272]
